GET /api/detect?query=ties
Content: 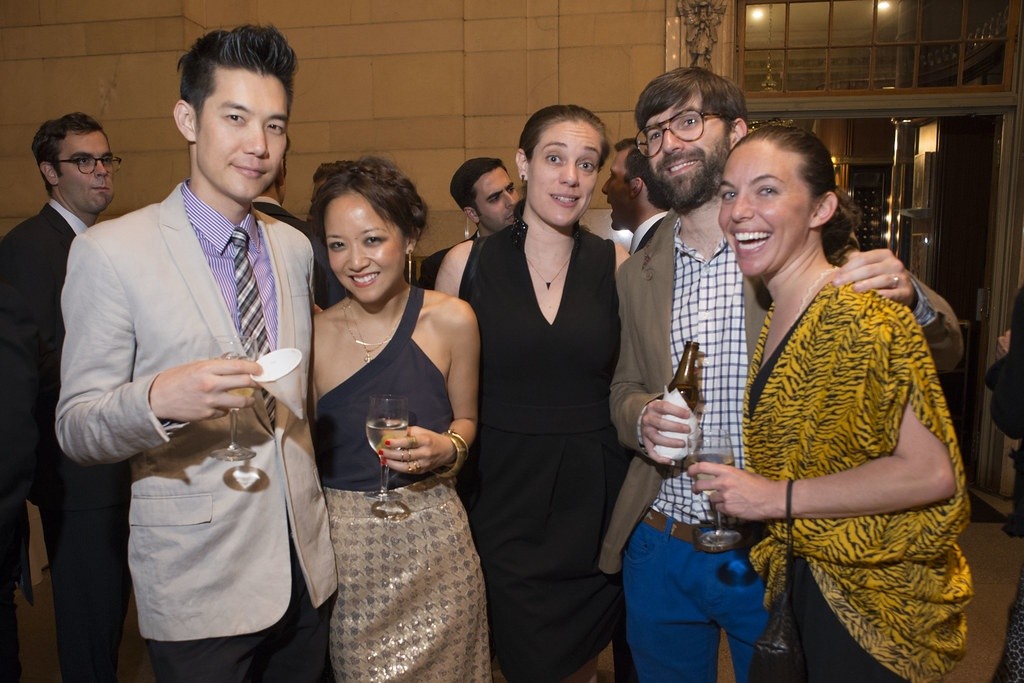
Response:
[230,225,276,427]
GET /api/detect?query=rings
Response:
[401,448,411,462]
[407,460,421,474]
[405,435,417,448]
[891,274,899,288]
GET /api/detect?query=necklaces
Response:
[341,285,410,363]
[527,257,570,290]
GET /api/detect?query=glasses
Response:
[634,108,735,159]
[50,156,122,175]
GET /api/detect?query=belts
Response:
[641,506,760,553]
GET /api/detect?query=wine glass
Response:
[365,392,410,501]
[208,335,258,462]
[687,427,743,547]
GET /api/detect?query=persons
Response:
[54,21,340,683]
[983,285,1024,683]
[248,133,307,235]
[308,159,493,683]
[422,157,520,291]
[436,106,628,683]
[602,138,669,255]
[599,67,963,683]
[688,126,973,683]
[0,113,135,683]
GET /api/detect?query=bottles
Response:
[666,340,700,412]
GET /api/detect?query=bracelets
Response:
[431,428,469,477]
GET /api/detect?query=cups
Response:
[250,348,305,422]
